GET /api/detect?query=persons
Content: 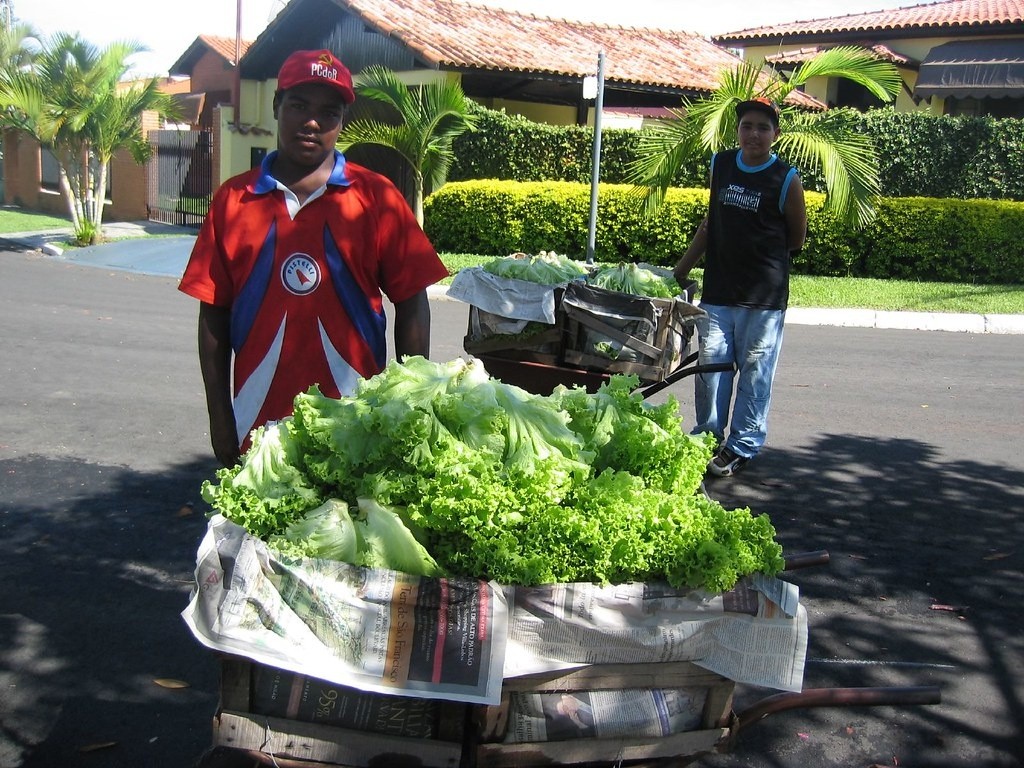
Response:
[177,50,448,465]
[674,97,806,478]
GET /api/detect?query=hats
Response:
[734,99,780,126]
[276,51,355,107]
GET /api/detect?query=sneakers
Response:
[711,444,748,479]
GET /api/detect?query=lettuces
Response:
[482,250,683,298]
[200,356,786,594]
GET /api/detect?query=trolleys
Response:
[212,541,941,768]
[462,279,737,400]
